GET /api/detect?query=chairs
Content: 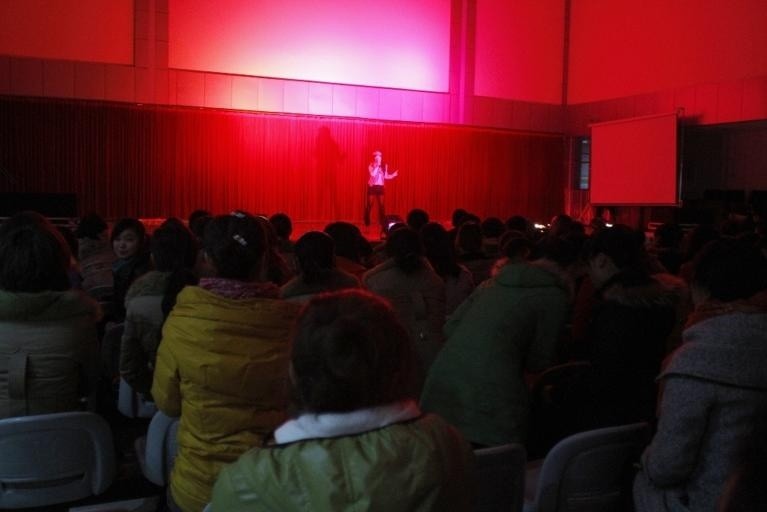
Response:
[469,361,656,511]
[1,378,181,510]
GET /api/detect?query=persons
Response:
[364,151,399,234]
